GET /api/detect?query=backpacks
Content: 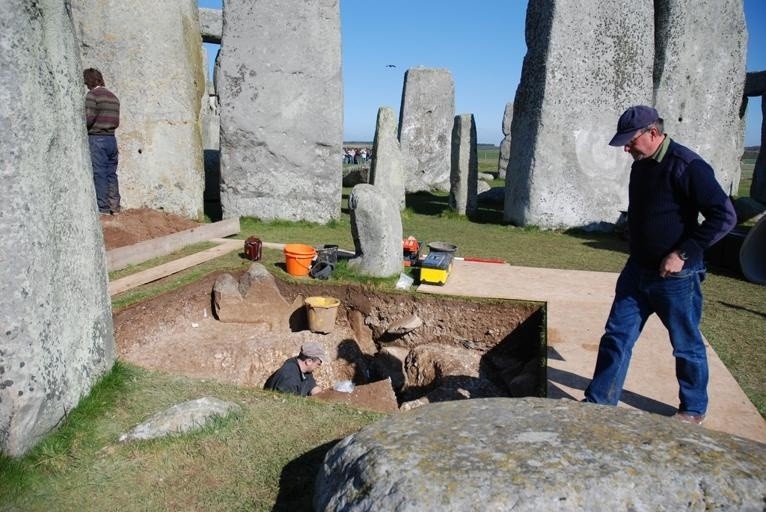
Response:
[244,236,263,262]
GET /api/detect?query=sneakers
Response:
[670,411,707,424]
[98,209,120,216]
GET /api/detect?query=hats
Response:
[609,105,658,147]
[311,261,332,279]
[301,341,331,363]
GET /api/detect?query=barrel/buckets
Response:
[284,243,315,276]
[306,295,340,334]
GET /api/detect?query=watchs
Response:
[677,248,690,261]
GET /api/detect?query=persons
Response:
[80,67,122,217]
[342,147,372,164]
[578,107,739,428]
[262,340,327,400]
